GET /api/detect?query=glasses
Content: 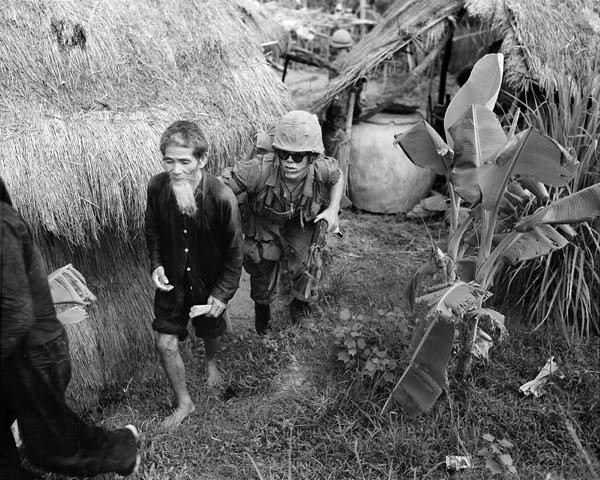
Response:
[277,152,308,163]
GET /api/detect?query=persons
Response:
[219,109,344,334]
[0,176,142,480]
[144,119,244,429]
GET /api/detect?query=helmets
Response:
[329,31,353,48]
[271,110,325,153]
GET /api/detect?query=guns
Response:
[307,129,352,268]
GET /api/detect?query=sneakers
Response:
[289,300,315,328]
[124,426,141,472]
[253,304,270,336]
